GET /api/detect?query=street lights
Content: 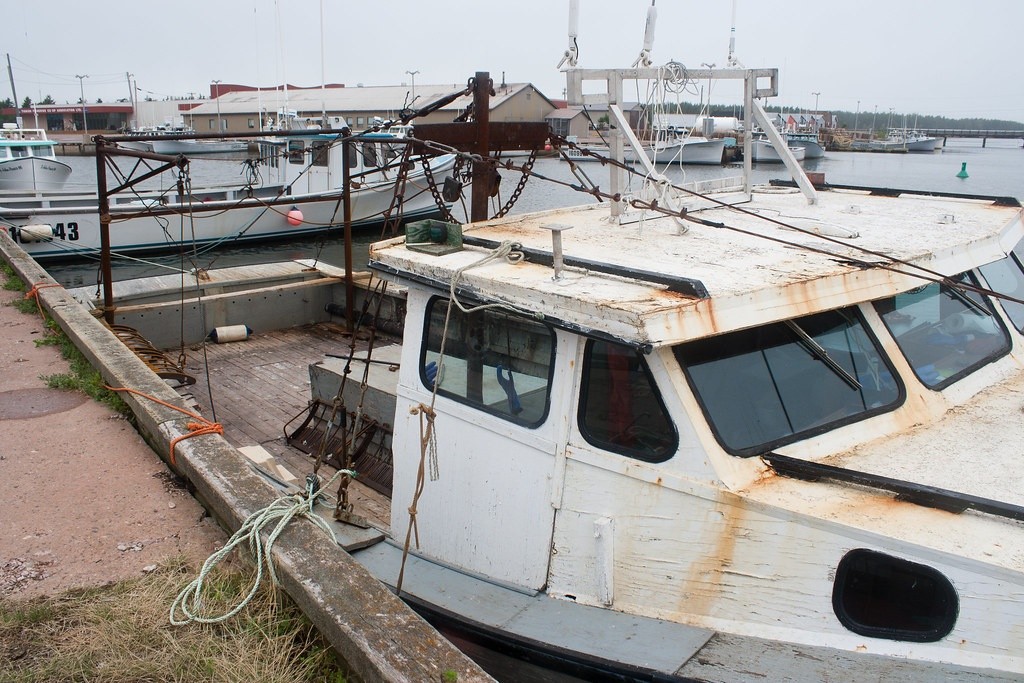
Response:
[700,62,716,118]
[209,79,224,133]
[404,71,421,124]
[811,92,821,134]
[75,74,91,134]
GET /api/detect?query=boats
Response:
[1,114,73,190]
[0,1,464,263]
[557,125,727,166]
[113,81,415,164]
[751,110,945,163]
[65,1,1022,683]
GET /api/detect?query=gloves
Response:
[497,365,521,413]
[425,361,438,385]
